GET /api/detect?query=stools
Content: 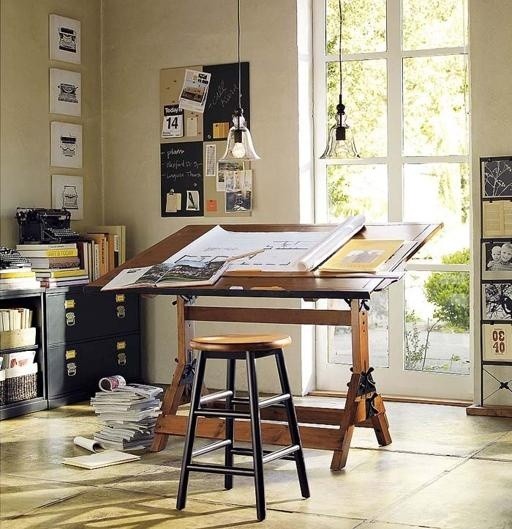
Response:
[174,333,313,522]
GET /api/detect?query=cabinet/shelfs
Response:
[0,292,47,419]
[47,330,142,410]
[46,285,140,346]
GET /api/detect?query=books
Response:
[60,373,165,470]
[1,308,38,375]
[99,257,230,293]
[0,222,126,294]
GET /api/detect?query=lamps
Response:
[319,0,364,163]
[219,0,260,165]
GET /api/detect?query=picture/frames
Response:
[475,147,512,407]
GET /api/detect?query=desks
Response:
[83,222,448,471]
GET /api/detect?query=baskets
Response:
[0,362,39,406]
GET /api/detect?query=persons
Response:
[486,244,502,268]
[486,242,512,271]
